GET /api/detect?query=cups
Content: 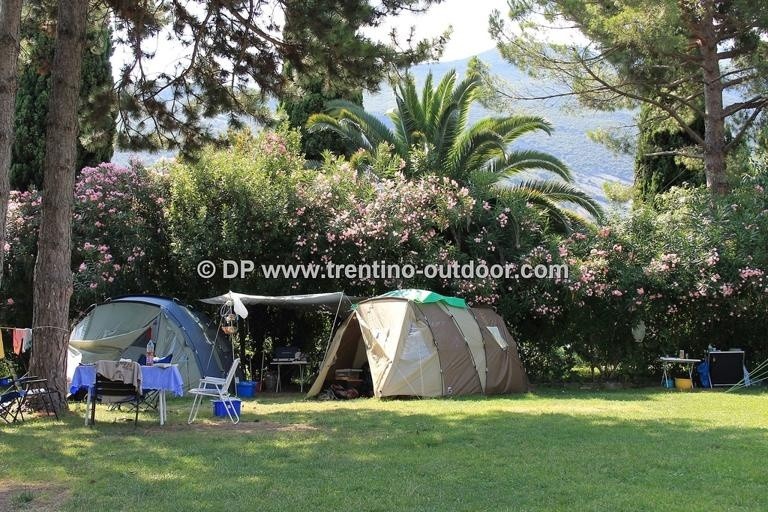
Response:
[713,348,716,352]
[680,350,684,358]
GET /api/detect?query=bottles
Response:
[664,377,674,388]
[147,339,155,365]
[708,344,712,352]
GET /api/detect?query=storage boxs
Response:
[237,381,256,398]
[210,398,242,417]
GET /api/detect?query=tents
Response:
[62,289,234,400]
[310,289,529,398]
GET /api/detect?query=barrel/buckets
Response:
[664,376,674,389]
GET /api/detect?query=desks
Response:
[270,360,313,394]
[660,357,702,390]
[703,350,748,385]
[71,363,184,427]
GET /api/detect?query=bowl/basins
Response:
[675,378,692,390]
[222,314,238,334]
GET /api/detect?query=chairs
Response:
[188,358,241,426]
[90,354,174,427]
[0,373,61,425]
